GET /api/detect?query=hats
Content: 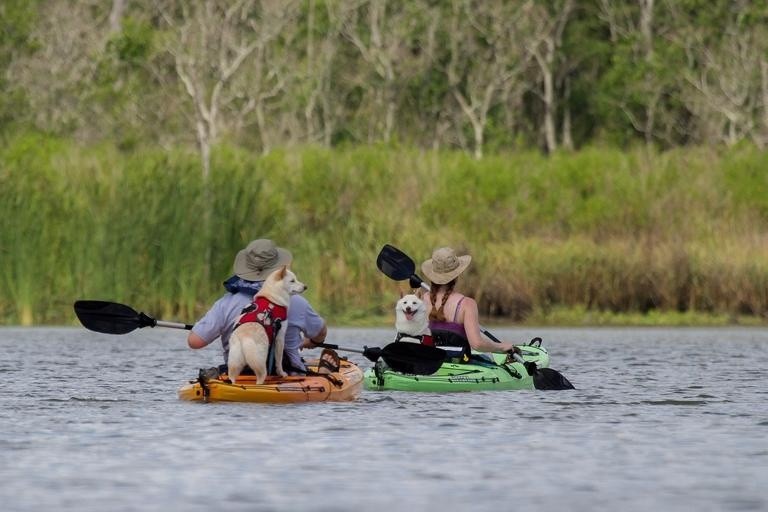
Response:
[233,239,292,282]
[422,247,471,287]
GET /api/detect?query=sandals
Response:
[318,348,341,374]
[505,347,522,363]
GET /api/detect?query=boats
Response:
[179,357,363,404]
[365,337,550,392]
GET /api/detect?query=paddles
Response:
[72,300,445,376]
[376,244,575,391]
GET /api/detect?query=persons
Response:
[408,246,515,365]
[187,239,341,375]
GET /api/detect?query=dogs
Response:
[227,264,308,384]
[394,286,436,345]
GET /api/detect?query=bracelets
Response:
[310,338,327,347]
